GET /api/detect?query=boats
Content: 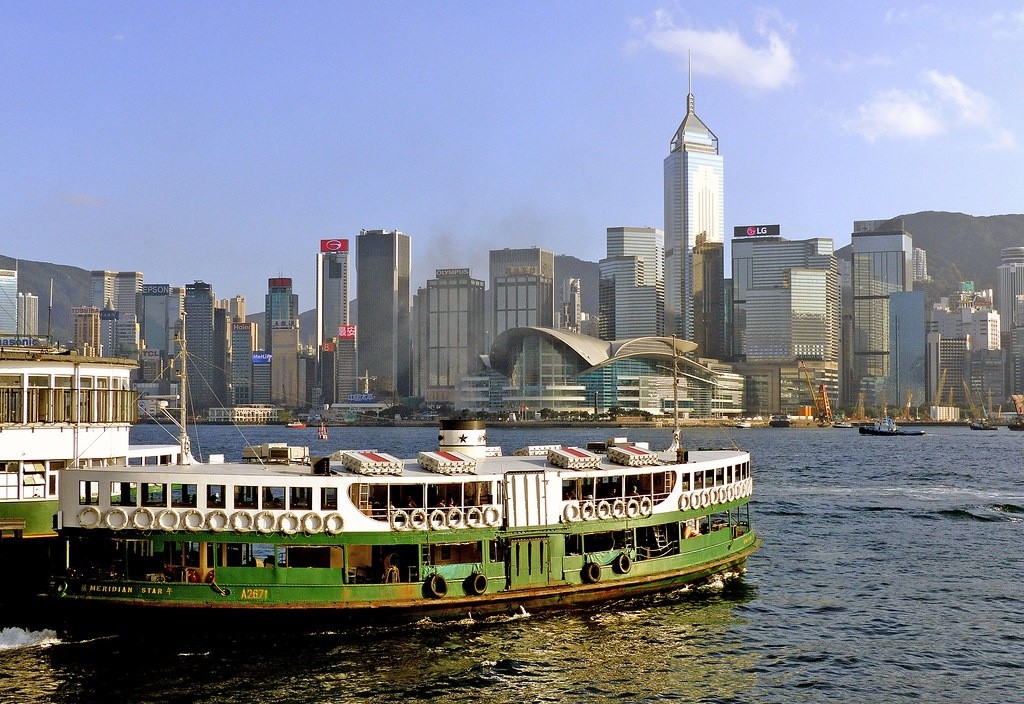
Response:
[969,418,999,431]
[767,413,791,427]
[41,312,760,630]
[284,421,306,428]
[832,421,853,428]
[735,422,751,428]
[859,422,925,436]
[317,423,328,440]
[1006,418,1024,431]
[0,352,204,540]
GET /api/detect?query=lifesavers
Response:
[581,562,602,585]
[76,505,347,536]
[427,574,448,598]
[469,573,488,596]
[613,553,632,576]
[563,495,653,524]
[679,477,754,511]
[391,503,503,531]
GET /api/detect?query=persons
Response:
[439,499,446,507]
[632,486,639,496]
[385,501,395,516]
[607,487,616,503]
[450,498,457,507]
[407,496,416,515]
